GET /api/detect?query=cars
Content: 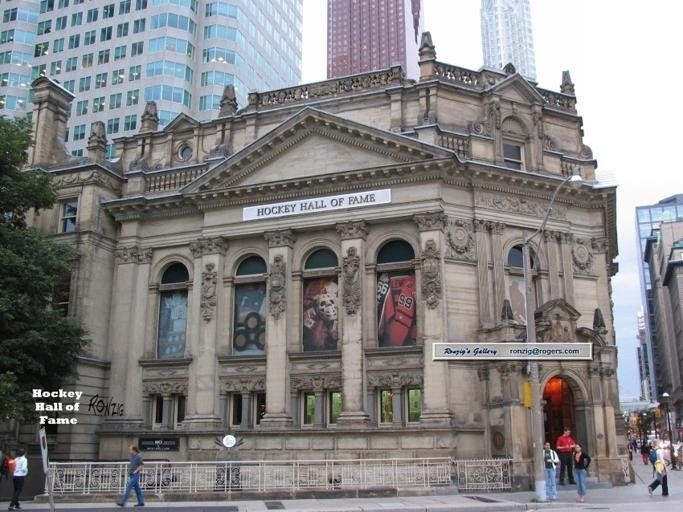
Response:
[675,442,683,467]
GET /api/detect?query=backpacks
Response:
[650,449,657,464]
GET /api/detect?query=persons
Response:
[627,433,683,496]
[0,447,30,510]
[116,446,145,506]
[543,441,560,499]
[556,426,576,486]
[573,444,592,502]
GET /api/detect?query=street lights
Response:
[638,392,677,470]
[522,175,584,502]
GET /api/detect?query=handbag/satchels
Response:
[8,459,15,472]
